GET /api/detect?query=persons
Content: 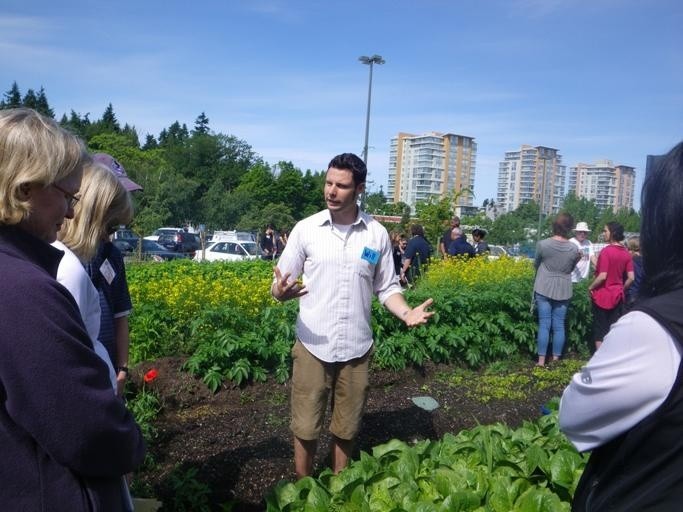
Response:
[53,162,137,399]
[568,220,599,283]
[557,138,682,510]
[625,234,646,304]
[588,221,636,352]
[1,107,147,512]
[256,215,491,288]
[84,152,143,398]
[266,151,436,485]
[532,212,577,368]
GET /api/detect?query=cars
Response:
[486,244,512,260]
[142,228,186,242]
[193,241,266,262]
[115,238,185,262]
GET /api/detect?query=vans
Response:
[212,230,258,243]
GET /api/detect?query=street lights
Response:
[537,155,550,241]
[357,55,385,212]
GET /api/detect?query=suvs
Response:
[158,232,203,254]
[113,230,139,239]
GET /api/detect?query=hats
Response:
[573,222,591,232]
[92,152,143,192]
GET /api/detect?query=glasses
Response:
[54,183,82,208]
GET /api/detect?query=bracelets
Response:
[116,366,130,378]
[270,283,282,303]
